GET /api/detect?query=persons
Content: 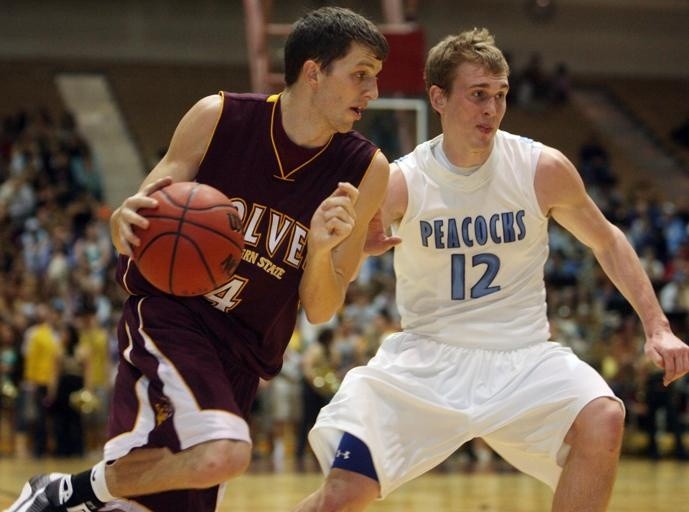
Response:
[251,243,395,468]
[2,6,391,512]
[1,107,127,460]
[541,132,689,462]
[502,52,570,110]
[285,25,689,512]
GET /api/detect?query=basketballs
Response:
[129,180,245,297]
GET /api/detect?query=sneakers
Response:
[5,472,73,511]
